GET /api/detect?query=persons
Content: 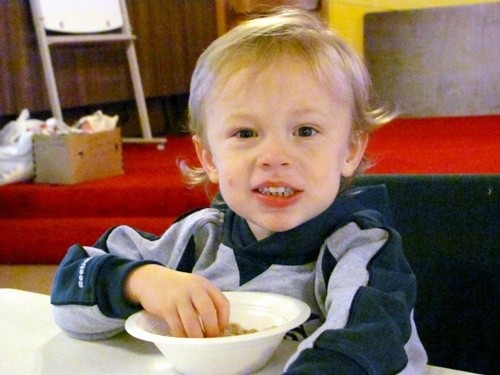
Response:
[50,4,429,375]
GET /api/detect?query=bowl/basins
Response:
[124,291,311,375]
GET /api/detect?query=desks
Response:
[0,287,478,375]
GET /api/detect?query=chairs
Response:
[213,172,499,375]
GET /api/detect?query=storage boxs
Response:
[31,126,122,185]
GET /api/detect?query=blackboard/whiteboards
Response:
[362,4,499,119]
